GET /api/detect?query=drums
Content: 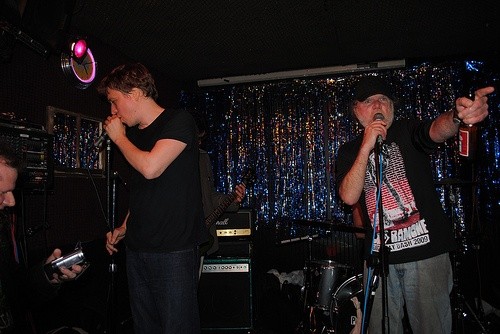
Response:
[299,257,355,316]
[329,272,376,334]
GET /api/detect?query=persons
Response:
[190,112,246,328]
[334,79,495,334]
[0,154,82,334]
[98,63,209,334]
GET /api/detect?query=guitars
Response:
[197,168,257,257]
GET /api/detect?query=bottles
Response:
[457,86,479,164]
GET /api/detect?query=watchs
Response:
[453,110,468,127]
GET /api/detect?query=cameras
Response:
[44,249,86,280]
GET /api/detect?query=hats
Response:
[354,76,395,102]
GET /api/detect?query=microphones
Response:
[87,131,108,156]
[374,113,385,147]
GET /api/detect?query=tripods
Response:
[440,183,488,334]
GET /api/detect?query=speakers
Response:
[197,257,254,330]
